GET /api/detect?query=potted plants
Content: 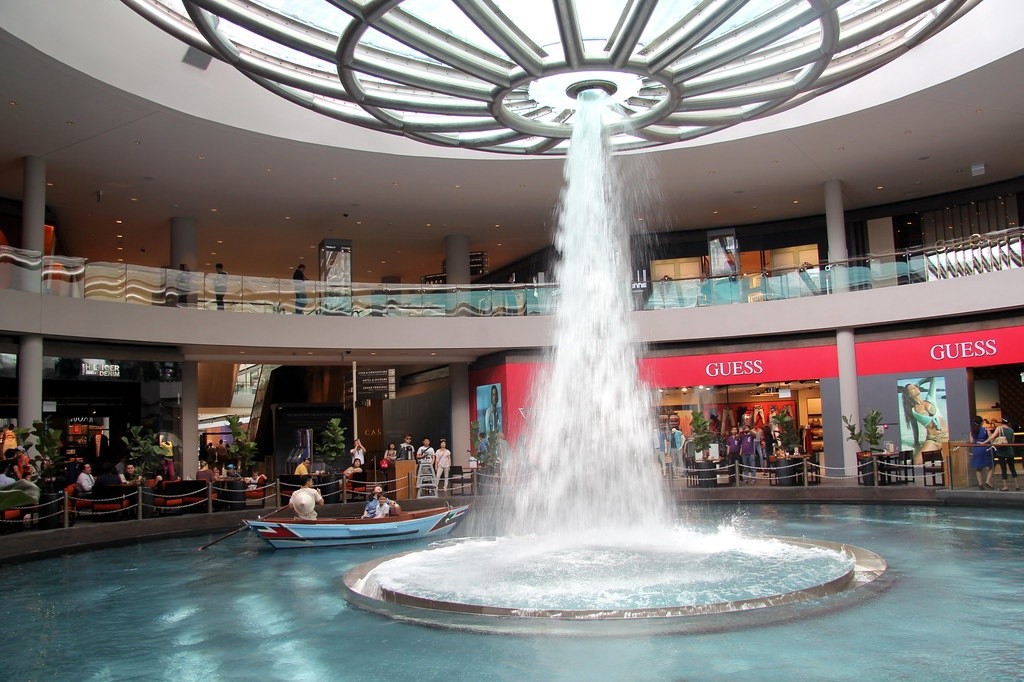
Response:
[470,417,500,493]
[14,416,67,530]
[840,408,886,486]
[120,422,167,518]
[689,410,718,487]
[215,413,259,511]
[767,409,800,486]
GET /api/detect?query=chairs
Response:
[89,482,139,521]
[887,447,915,486]
[797,452,821,485]
[920,449,945,486]
[766,455,779,486]
[855,451,885,485]
[685,456,699,488]
[280,472,319,507]
[343,470,367,501]
[449,464,472,496]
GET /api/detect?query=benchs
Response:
[143,474,208,519]
[62,475,97,519]
[312,415,347,504]
[244,474,268,508]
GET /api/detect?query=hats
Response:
[292,490,315,515]
[373,487,382,493]
[304,457,311,462]
[4,448,20,458]
[227,464,235,469]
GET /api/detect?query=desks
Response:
[872,450,901,485]
[463,466,483,470]
[778,454,811,460]
[694,458,722,464]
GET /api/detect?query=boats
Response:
[241,503,472,551]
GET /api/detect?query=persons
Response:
[969,415,997,490]
[288,473,323,521]
[763,425,776,456]
[160,436,175,481]
[485,385,499,439]
[214,263,228,311]
[0,436,451,534]
[738,425,757,483]
[668,427,687,477]
[496,432,510,464]
[293,263,310,314]
[175,263,190,307]
[977,415,1021,491]
[652,429,666,478]
[902,376,949,463]
[725,425,743,482]
[478,433,490,459]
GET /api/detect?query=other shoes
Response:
[1001,488,1009,491]
[979,484,986,490]
[1016,488,1020,491]
[986,481,995,490]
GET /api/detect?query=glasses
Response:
[407,438,412,440]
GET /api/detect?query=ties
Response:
[89,475,94,484]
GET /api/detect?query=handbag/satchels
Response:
[380,460,388,468]
[991,427,1008,444]
